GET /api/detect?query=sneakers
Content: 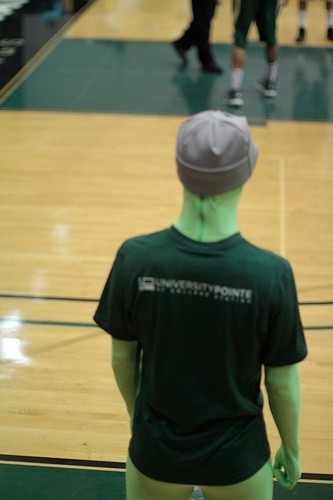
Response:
[255,80,277,98]
[224,90,244,105]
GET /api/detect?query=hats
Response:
[175,110,259,194]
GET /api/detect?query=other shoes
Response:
[327,29,333,41]
[296,30,305,41]
[202,65,222,73]
[172,41,186,59]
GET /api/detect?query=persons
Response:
[92,111,308,500]
[172,0,223,74]
[295,0,333,42]
[224,0,289,105]
